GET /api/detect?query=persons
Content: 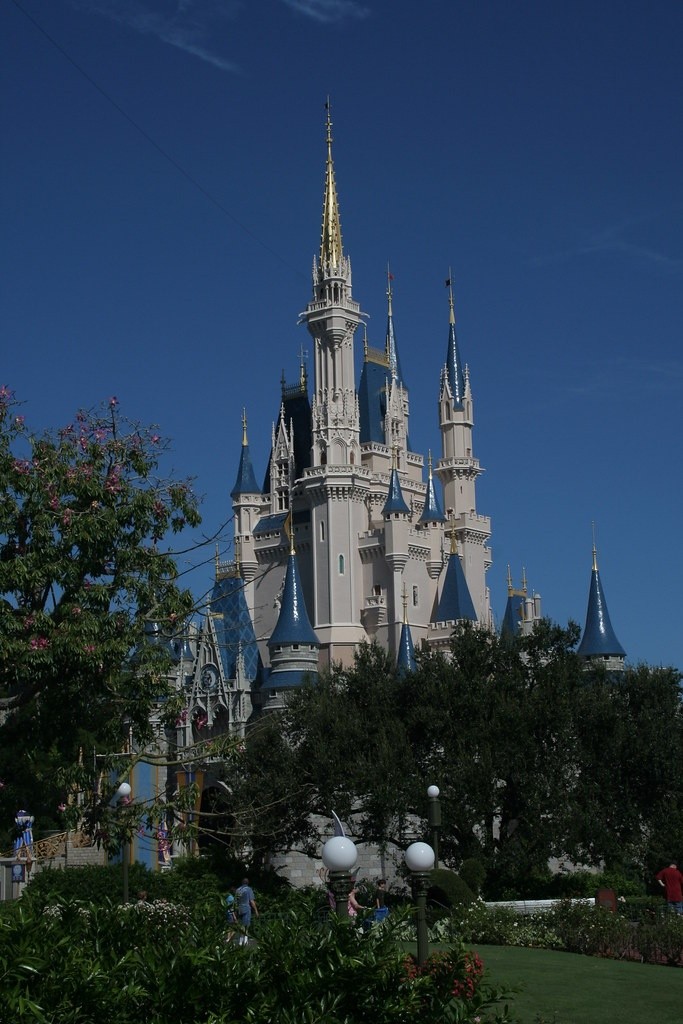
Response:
[236,878,259,947]
[347,886,365,917]
[655,861,683,916]
[224,888,239,942]
[375,879,386,909]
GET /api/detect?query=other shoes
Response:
[239,938,243,946]
[244,935,249,947]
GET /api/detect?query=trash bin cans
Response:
[594,887,618,913]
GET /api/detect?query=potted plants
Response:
[584,872,641,913]
